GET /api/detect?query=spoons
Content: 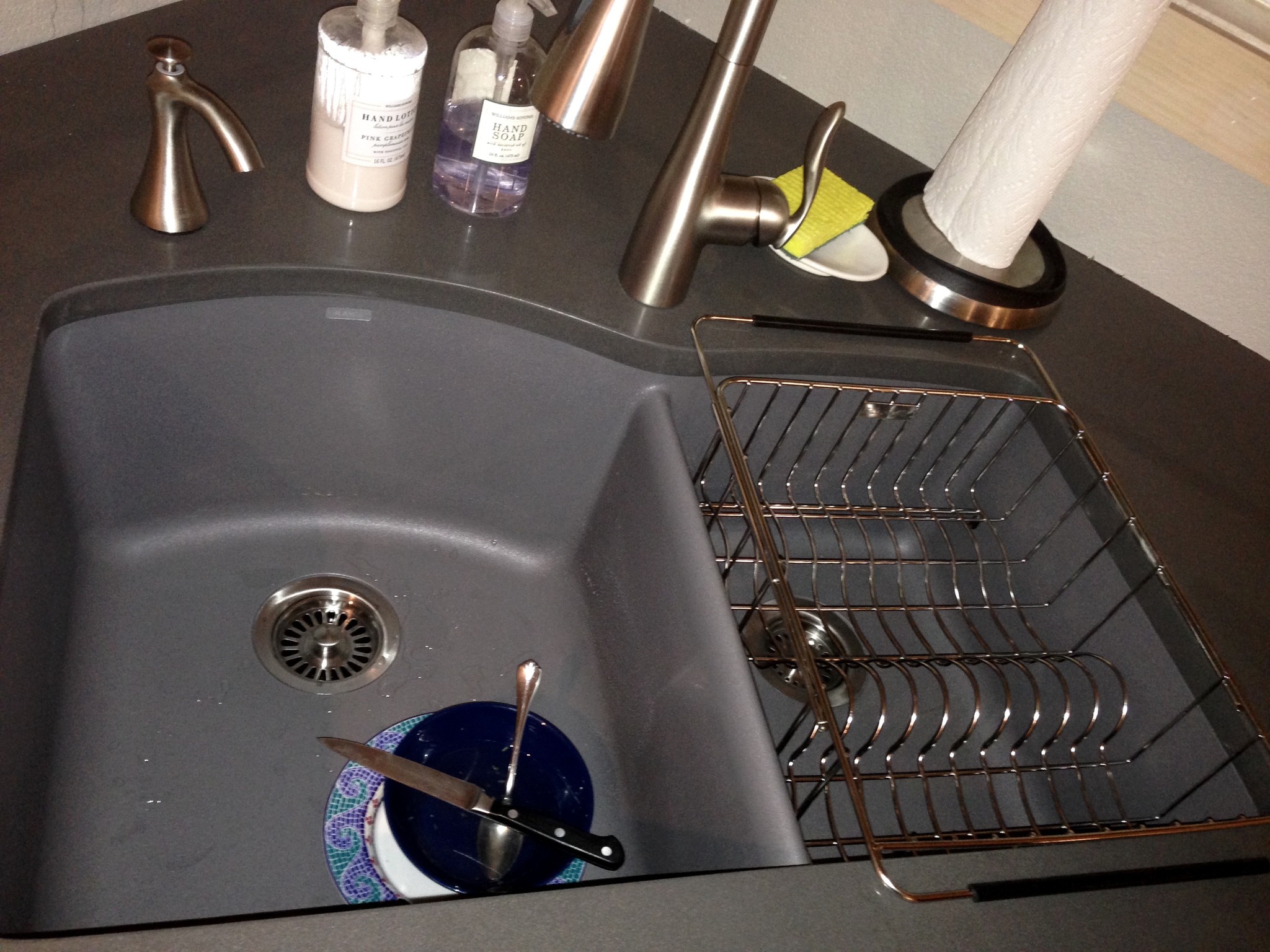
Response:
[476,660,543,880]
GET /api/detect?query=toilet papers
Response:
[923,2,1176,276]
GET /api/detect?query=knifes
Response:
[316,737,625,871]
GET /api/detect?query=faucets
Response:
[524,0,849,312]
[122,37,265,236]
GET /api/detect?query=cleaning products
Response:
[304,2,433,218]
[433,0,558,221]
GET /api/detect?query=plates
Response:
[745,176,889,282]
[323,701,594,905]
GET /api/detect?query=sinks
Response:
[648,344,1267,868]
[0,258,810,940]
